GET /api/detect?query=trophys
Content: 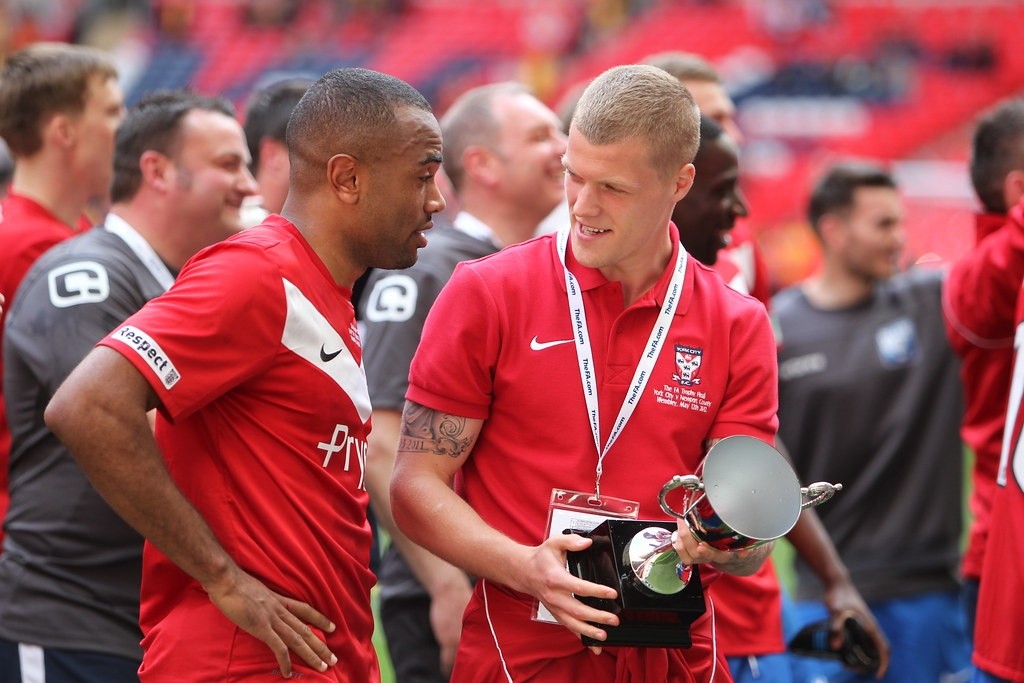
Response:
[562,434,843,649]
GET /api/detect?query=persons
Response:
[672,111,889,683]
[636,53,769,315]
[389,65,779,683]
[944,102,1024,683]
[44,67,447,683]
[0,89,260,683]
[238,78,316,228]
[357,81,568,683]
[0,41,129,558]
[768,164,963,683]
